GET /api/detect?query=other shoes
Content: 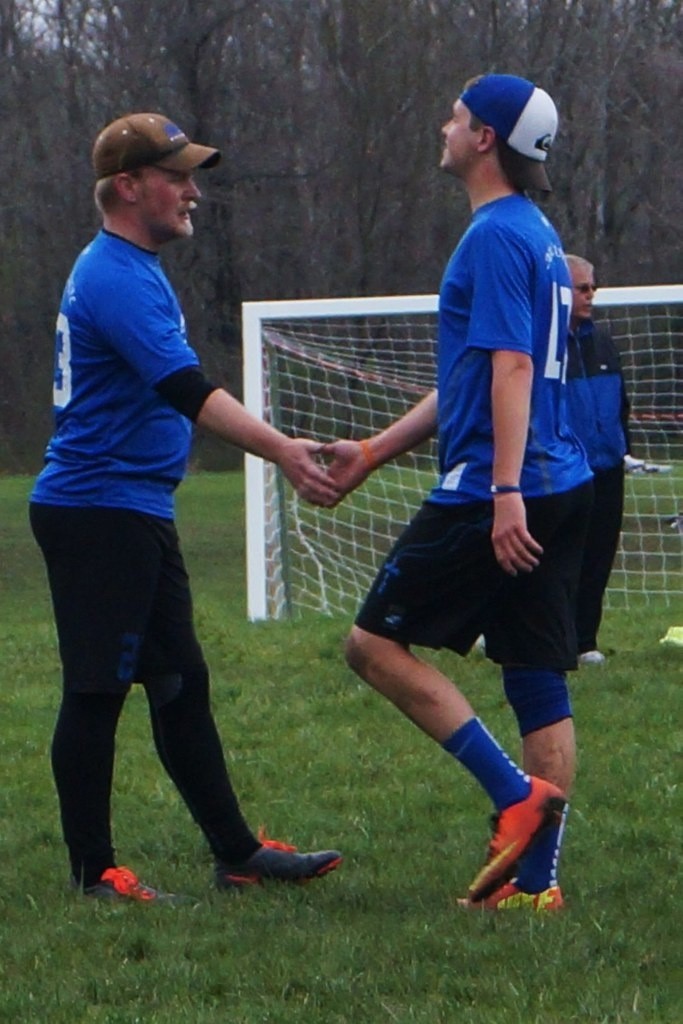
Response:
[578,651,604,663]
[475,635,485,650]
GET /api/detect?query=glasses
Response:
[570,282,596,294]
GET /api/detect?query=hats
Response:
[460,73,558,192]
[93,112,221,178]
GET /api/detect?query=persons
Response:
[30,113,344,905]
[323,73,597,913]
[479,250,632,665]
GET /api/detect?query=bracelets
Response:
[489,484,523,494]
[360,439,376,471]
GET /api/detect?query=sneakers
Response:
[212,826,342,888]
[467,776,567,905]
[74,864,197,905]
[456,880,562,914]
